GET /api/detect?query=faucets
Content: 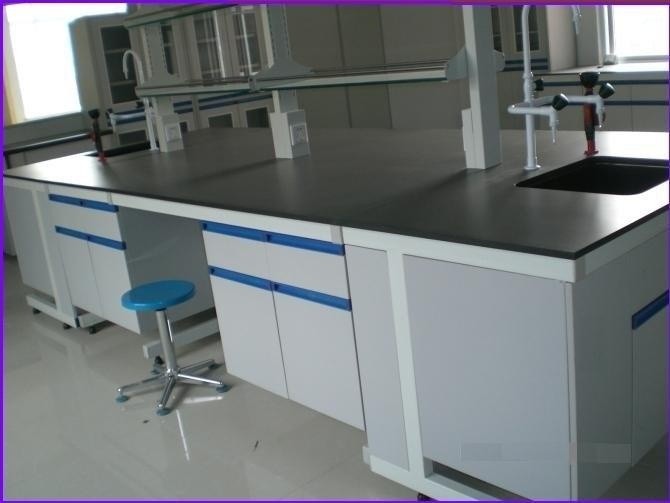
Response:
[535,82,616,128]
[123,50,160,150]
[105,107,146,134]
[520,4,583,171]
[507,92,569,144]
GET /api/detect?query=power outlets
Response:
[290,122,308,146]
[165,124,182,142]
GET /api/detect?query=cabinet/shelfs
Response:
[200,220,367,433]
[43,192,214,337]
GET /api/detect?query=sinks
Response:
[83,140,159,158]
[514,155,670,196]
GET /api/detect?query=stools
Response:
[115,280,229,416]
